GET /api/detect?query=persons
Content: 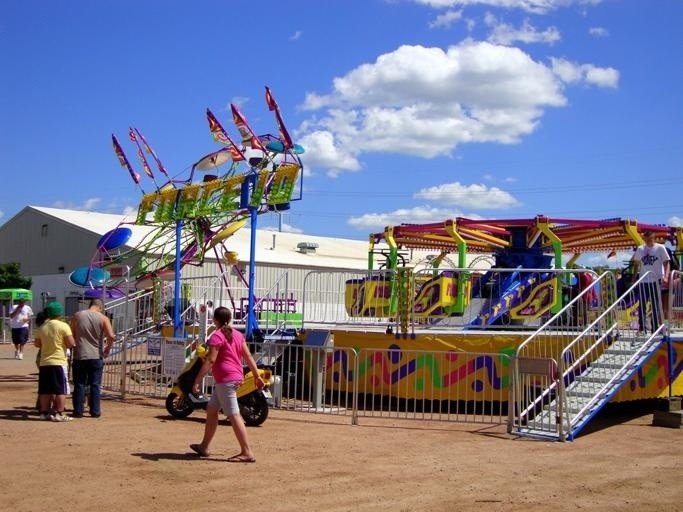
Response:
[190,306,265,462]
[9,298,33,360]
[35,301,76,422]
[630,231,670,335]
[34,311,68,413]
[70,298,114,418]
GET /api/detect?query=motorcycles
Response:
[165,337,273,429]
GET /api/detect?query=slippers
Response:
[189,444,210,457]
[228,455,256,463]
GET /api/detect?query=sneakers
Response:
[39,409,51,421]
[14,349,24,360]
[49,411,74,422]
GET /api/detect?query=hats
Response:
[46,300,64,317]
